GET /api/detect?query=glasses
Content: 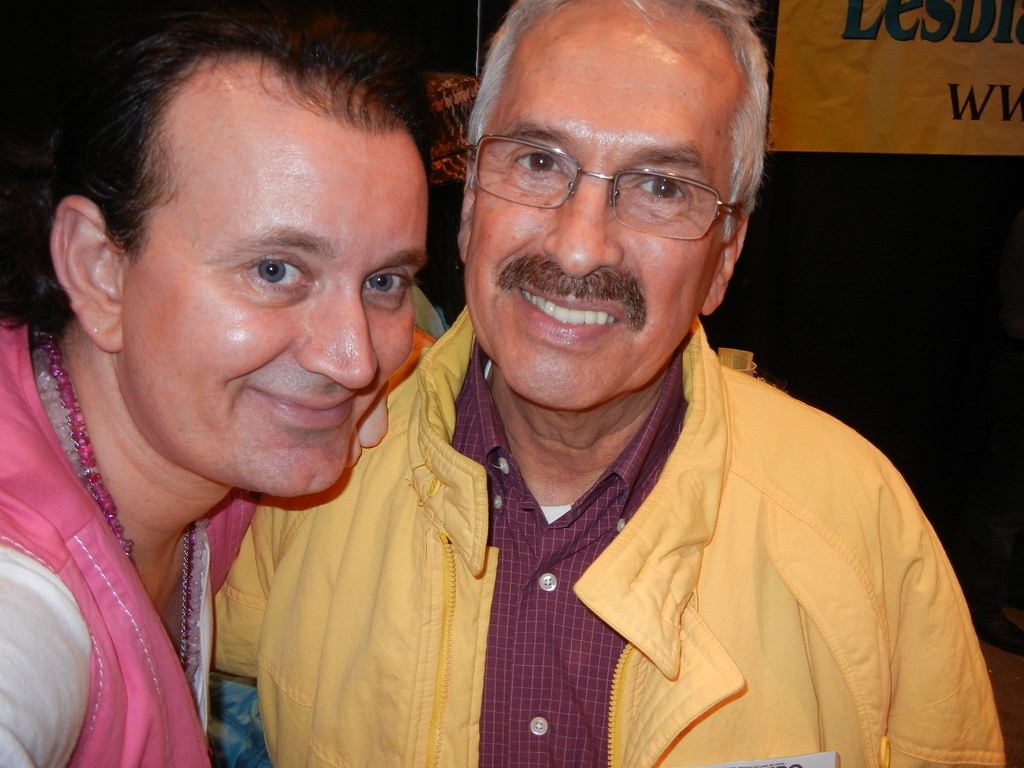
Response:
[467,134,743,240]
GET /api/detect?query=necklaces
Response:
[41,335,196,677]
[31,349,207,686]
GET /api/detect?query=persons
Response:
[2,0,428,767]
[213,1,1005,767]
